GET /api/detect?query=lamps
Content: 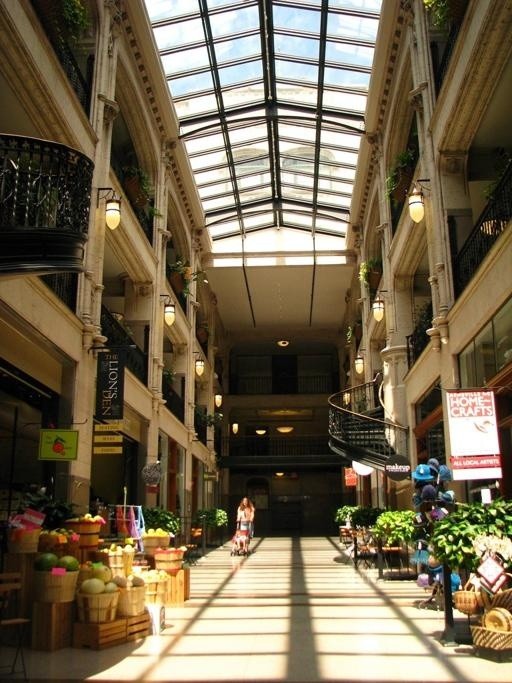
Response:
[372,289,388,322]
[160,294,175,327]
[353,348,366,374]
[96,187,121,233]
[192,351,204,377]
[404,179,431,223]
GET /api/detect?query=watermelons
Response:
[58,556,79,571]
[33,553,58,570]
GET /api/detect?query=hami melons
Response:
[77,565,117,594]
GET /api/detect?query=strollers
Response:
[230,520,253,557]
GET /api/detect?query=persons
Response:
[237,496,255,552]
[234,529,246,551]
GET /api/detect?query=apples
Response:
[53,442,64,453]
